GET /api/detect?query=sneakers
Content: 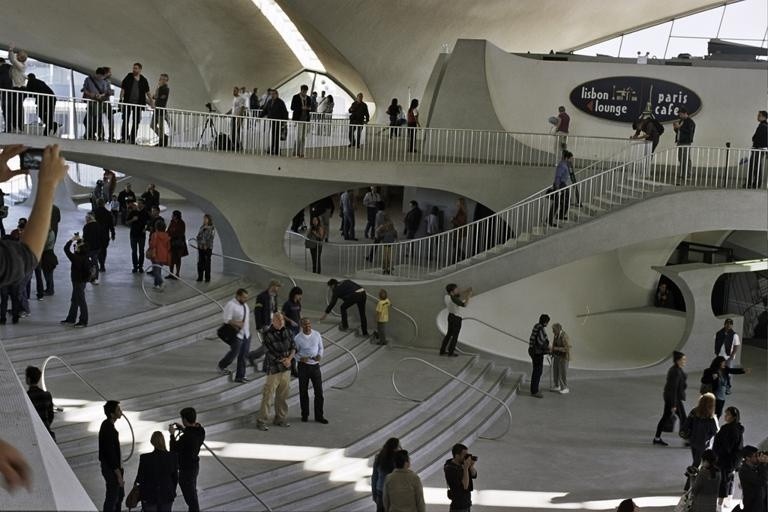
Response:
[257,423,269,430]
[531,392,543,398]
[550,386,561,391]
[234,375,249,382]
[217,365,231,374]
[274,421,291,427]
[559,389,569,394]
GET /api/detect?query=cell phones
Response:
[20,148,51,170]
[172,423,179,429]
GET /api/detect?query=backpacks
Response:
[647,118,664,135]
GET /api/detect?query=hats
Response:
[725,318,733,324]
[673,350,684,361]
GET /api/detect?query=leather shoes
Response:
[449,350,457,356]
[315,418,328,423]
[653,438,668,446]
[440,351,448,355]
[685,442,692,446]
[302,417,307,421]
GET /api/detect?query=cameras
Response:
[205,102,212,110]
[74,231,83,244]
[465,454,477,461]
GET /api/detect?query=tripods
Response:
[196,111,218,150]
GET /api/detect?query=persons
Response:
[686,393,719,491]
[103,167,117,195]
[0,144,70,326]
[363,186,380,237]
[83,212,103,284]
[546,151,574,227]
[26,73,62,136]
[16,223,43,301]
[655,351,686,445]
[739,445,768,512]
[147,220,171,291]
[561,143,583,207]
[291,85,312,157]
[388,98,401,139]
[317,198,335,242]
[261,89,288,156]
[126,199,152,275]
[283,287,305,331]
[348,93,369,149]
[549,106,570,155]
[371,438,406,512]
[167,210,188,279]
[0,439,34,489]
[687,449,720,512]
[256,311,296,431]
[407,99,421,153]
[97,67,117,143]
[249,87,263,127]
[109,195,120,223]
[0,57,12,133]
[305,217,325,274]
[259,88,271,118]
[702,356,750,419]
[240,87,249,130]
[444,443,477,512]
[83,66,108,141]
[675,109,696,178]
[630,115,664,165]
[169,408,205,512]
[401,201,421,259]
[119,183,136,224]
[146,207,161,274]
[324,94,334,136]
[216,289,252,383]
[223,87,245,152]
[18,218,27,225]
[92,180,103,208]
[743,111,768,188]
[713,406,743,511]
[423,207,440,260]
[438,282,473,356]
[0,284,20,324]
[64,234,92,327]
[383,449,426,512]
[149,72,169,146]
[528,314,552,397]
[25,366,54,435]
[134,432,178,512]
[549,323,572,394]
[294,318,328,424]
[377,218,398,275]
[196,213,214,281]
[95,198,115,272]
[364,201,387,261]
[616,498,640,512]
[118,63,154,144]
[8,41,27,134]
[450,199,467,265]
[248,279,284,370]
[317,91,328,135]
[396,104,406,131]
[714,319,741,394]
[342,188,358,241]
[10,229,30,319]
[320,278,369,336]
[310,91,318,114]
[39,225,59,295]
[99,401,124,512]
[290,208,305,233]
[373,289,390,345]
[142,184,161,207]
[52,204,62,240]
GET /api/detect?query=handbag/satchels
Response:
[216,302,246,343]
[700,369,711,394]
[662,414,677,432]
[545,188,562,201]
[291,358,300,377]
[306,234,316,248]
[146,248,155,258]
[675,488,692,512]
[126,480,141,509]
[86,253,99,282]
[534,327,550,354]
